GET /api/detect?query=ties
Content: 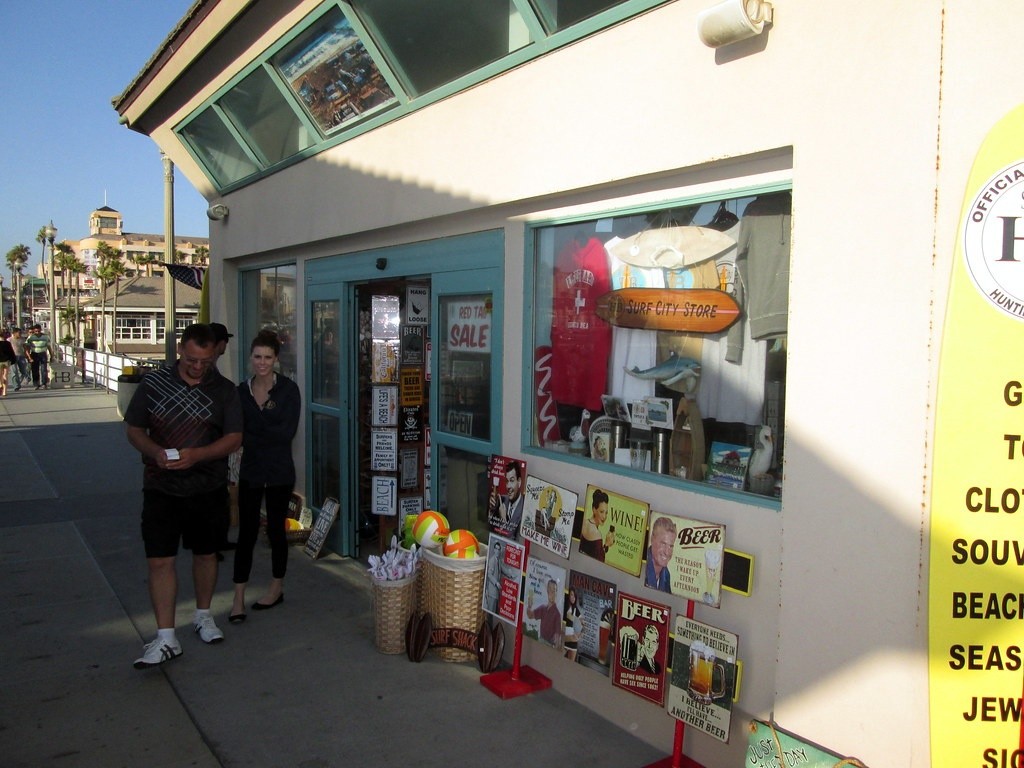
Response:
[508,505,513,519]
[497,557,498,571]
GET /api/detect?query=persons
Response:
[646,518,676,594]
[527,580,561,648]
[578,489,616,564]
[228,329,302,622]
[0,324,55,397]
[122,323,243,669]
[483,542,517,613]
[562,584,586,661]
[489,461,525,533]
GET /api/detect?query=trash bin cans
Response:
[117,375,141,419]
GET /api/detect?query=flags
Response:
[163,263,206,290]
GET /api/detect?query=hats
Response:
[208,323,234,339]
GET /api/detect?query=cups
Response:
[619,630,643,671]
[687,645,725,706]
[702,551,720,603]
[598,622,611,665]
[490,476,500,509]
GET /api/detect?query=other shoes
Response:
[228,610,247,624]
[251,591,284,611]
[34,386,39,390]
[15,385,21,392]
[42,384,47,389]
[0,386,3,396]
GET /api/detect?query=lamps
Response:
[697,0,772,48]
[206,204,229,221]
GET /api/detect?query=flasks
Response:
[652,427,670,473]
[610,420,628,463]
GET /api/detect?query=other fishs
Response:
[621,352,702,394]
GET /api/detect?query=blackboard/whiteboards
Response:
[302,497,341,560]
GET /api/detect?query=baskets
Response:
[258,524,313,545]
[373,540,488,663]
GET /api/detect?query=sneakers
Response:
[133,640,183,669]
[192,613,225,645]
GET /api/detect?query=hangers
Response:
[574,224,589,247]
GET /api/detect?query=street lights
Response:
[0,273,4,336]
[45,219,57,363]
[14,258,23,334]
